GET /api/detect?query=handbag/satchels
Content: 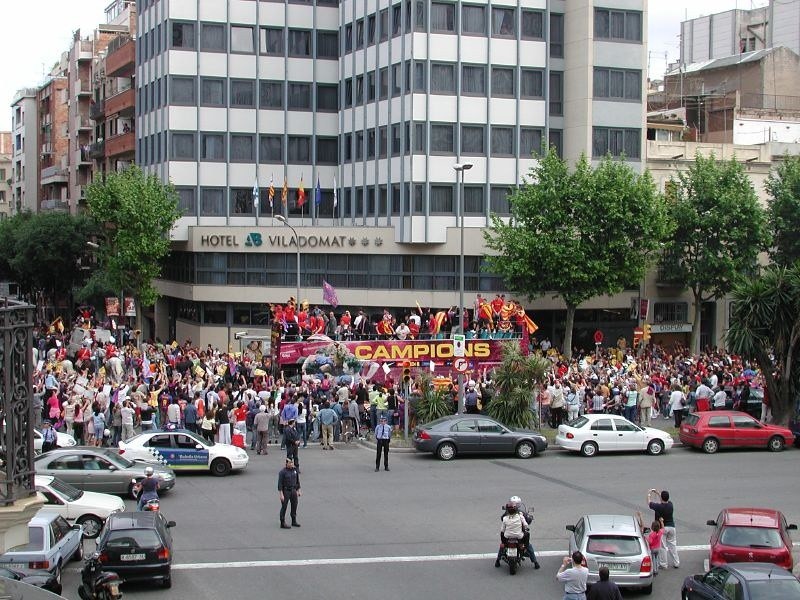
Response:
[681,392,686,406]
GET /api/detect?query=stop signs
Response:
[634,327,644,337]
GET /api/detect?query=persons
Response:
[278,458,301,528]
[586,566,623,600]
[80,123,130,152]
[375,417,391,471]
[557,550,589,600]
[495,496,540,569]
[648,489,680,568]
[647,518,665,575]
[284,419,301,473]
[316,402,338,450]
[137,467,160,512]
[29,295,780,454]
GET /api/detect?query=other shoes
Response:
[292,523,300,527]
[535,564,540,569]
[385,467,389,471]
[495,562,500,567]
[375,468,379,472]
[281,525,290,528]
[250,441,334,455]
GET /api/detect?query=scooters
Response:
[130,478,160,510]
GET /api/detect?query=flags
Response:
[46,316,64,335]
[253,170,338,208]
[323,280,340,308]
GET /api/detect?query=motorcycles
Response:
[77,537,124,600]
[499,503,536,575]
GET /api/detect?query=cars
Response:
[0,511,84,600]
[0,417,77,461]
[411,413,548,461]
[680,411,796,455]
[556,415,675,456]
[682,563,800,600]
[566,514,654,595]
[116,430,249,478]
[25,447,175,522]
[704,507,798,573]
[94,511,176,590]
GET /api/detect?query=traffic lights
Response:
[402,360,411,382]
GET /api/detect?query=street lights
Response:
[274,214,300,314]
[453,162,474,333]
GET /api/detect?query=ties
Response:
[382,426,384,435]
[45,429,48,442]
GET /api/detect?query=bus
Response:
[272,318,530,394]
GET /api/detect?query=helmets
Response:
[144,467,153,475]
[510,496,521,506]
[506,502,516,509]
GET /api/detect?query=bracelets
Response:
[561,564,565,568]
